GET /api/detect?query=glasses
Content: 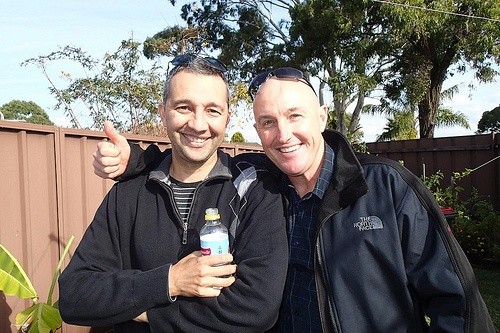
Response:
[248,66,318,100]
[167,53,230,83]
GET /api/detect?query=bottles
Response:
[200,208,231,290]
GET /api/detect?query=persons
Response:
[59,57,289,333]
[92,66,498,333]
